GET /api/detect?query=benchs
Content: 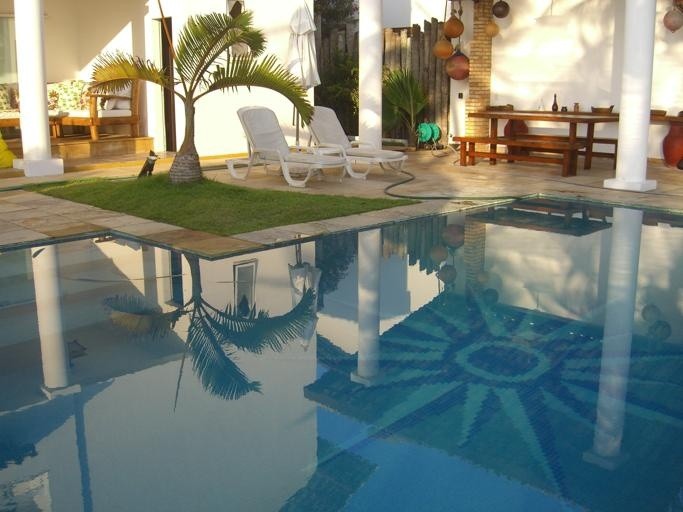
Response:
[496,134,618,170]
[452,135,584,177]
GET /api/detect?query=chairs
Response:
[304,106,408,179]
[225,105,348,188]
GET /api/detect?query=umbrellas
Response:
[286,241,322,351]
[283,2,321,146]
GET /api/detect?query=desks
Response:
[467,208,611,237]
[468,112,619,169]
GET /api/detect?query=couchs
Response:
[0,79,139,141]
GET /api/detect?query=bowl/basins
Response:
[650,110,666,116]
[590,106,614,114]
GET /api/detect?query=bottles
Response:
[551,94,580,113]
